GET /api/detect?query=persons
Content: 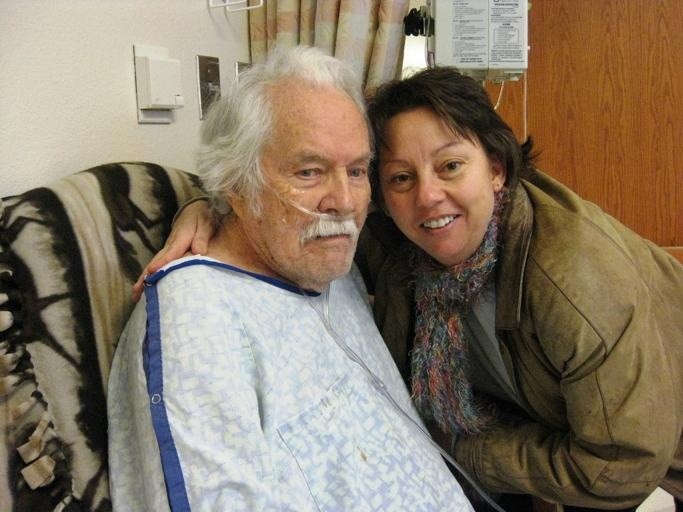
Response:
[129,65,683,511]
[101,41,478,511]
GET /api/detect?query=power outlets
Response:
[196,54,221,121]
